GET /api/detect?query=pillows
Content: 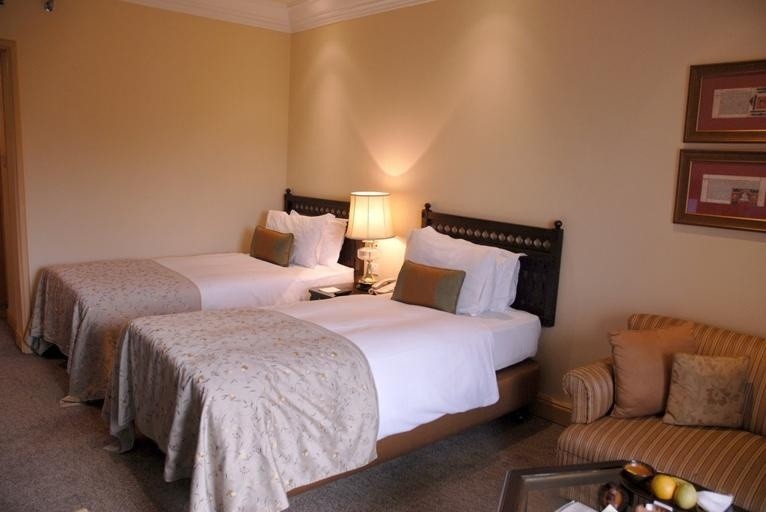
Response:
[606,320,698,421]
[248,207,348,268]
[660,351,754,430]
[388,224,529,317]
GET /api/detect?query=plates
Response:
[617,470,715,512]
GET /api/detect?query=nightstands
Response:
[308,283,369,301]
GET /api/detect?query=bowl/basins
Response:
[595,480,630,511]
[619,458,656,482]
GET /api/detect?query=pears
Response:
[674,481,699,509]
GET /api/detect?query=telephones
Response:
[370,277,397,294]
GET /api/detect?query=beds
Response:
[21,187,356,411]
[101,199,567,512]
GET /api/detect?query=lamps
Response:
[344,190,395,292]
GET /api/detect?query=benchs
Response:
[553,310,763,512]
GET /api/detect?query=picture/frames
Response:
[668,142,766,234]
[679,56,766,145]
